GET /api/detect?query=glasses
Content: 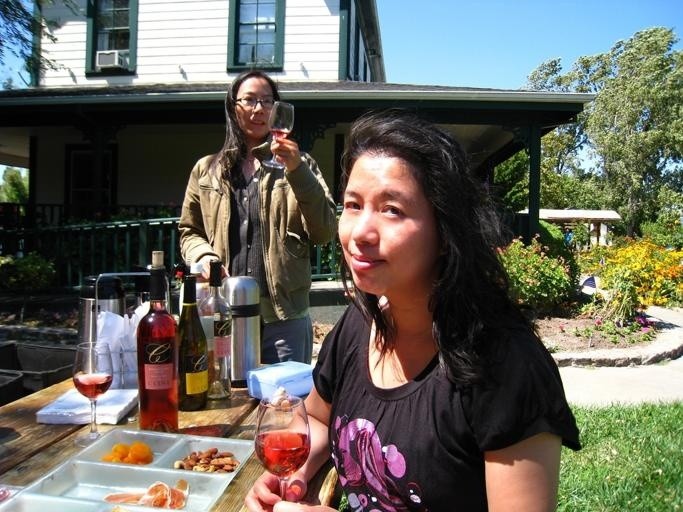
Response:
[236,97,275,108]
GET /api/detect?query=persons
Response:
[244,107,583,512]
[176,71,336,366]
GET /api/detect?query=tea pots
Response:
[76,273,126,374]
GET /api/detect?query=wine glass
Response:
[71,341,114,450]
[261,101,294,169]
[254,393,312,502]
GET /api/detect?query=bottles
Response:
[216,275,263,390]
[179,261,214,315]
[178,275,211,412]
[200,259,234,400]
[138,250,178,436]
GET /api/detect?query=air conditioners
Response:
[95,50,125,68]
